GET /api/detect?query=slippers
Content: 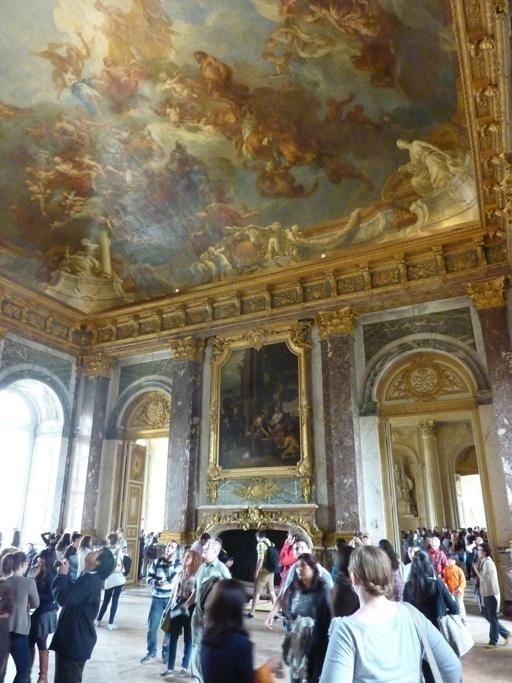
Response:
[260,540,281,572]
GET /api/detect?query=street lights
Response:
[169,596,191,625]
[435,577,476,657]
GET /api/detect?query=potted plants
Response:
[208,319,315,481]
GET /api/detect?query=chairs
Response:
[484,632,512,649]
[160,666,188,677]
[139,652,157,665]
[93,619,118,630]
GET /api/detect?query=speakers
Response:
[36,648,49,682]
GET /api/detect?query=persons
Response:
[248,405,300,461]
[0,525,131,683]
[392,464,403,500]
[138,525,509,683]
